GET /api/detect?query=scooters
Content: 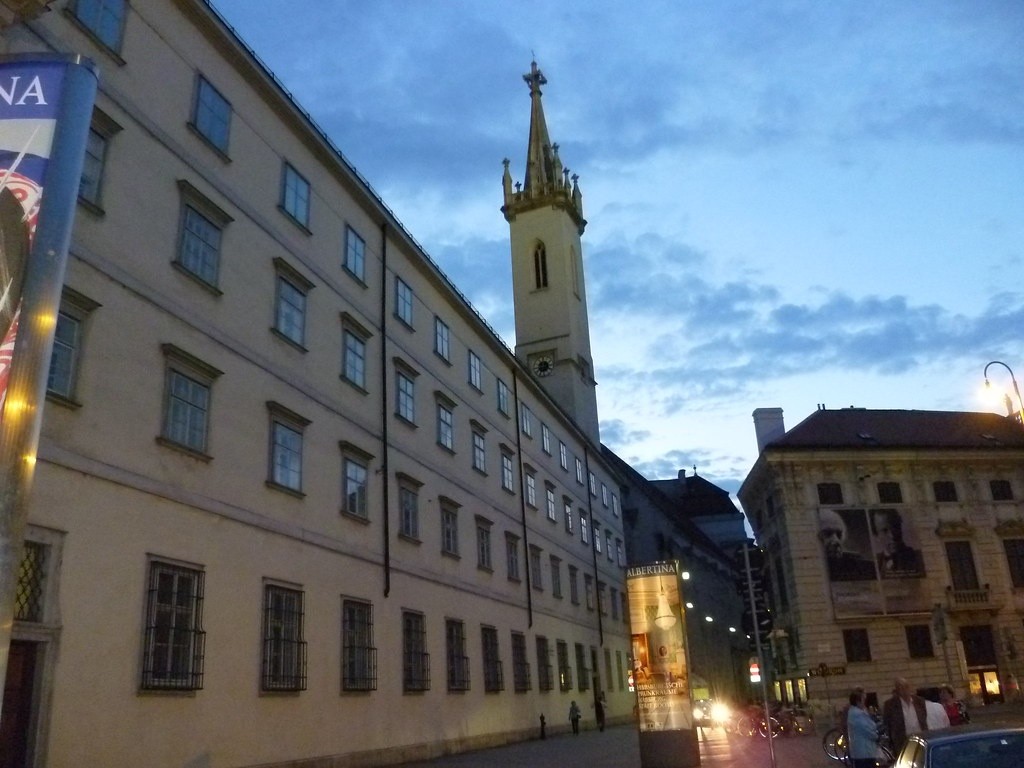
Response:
[890,682,972,725]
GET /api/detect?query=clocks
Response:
[533,355,555,378]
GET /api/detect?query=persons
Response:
[591,697,608,732]
[842,676,971,768]
[568,701,580,737]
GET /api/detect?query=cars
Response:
[890,725,1024,768]
[691,698,731,730]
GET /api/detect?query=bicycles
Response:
[822,715,887,761]
[834,727,886,766]
[724,701,813,740]
[842,745,895,768]
